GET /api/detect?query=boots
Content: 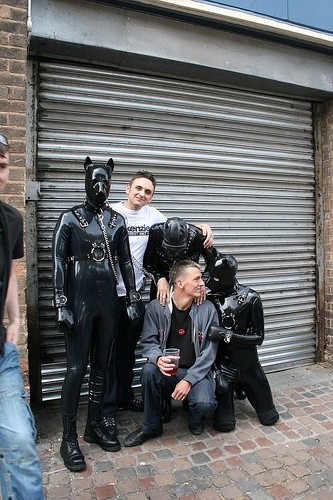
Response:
[160,395,172,423]
[234,381,247,399]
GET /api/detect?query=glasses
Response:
[0,134,11,149]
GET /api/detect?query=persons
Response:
[52,156,141,473]
[142,217,221,422]
[106,170,214,414]
[123,259,219,447]
[205,254,279,433]
[0,132,45,500]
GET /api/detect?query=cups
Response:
[163,348,180,375]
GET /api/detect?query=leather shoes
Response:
[188,416,205,435]
[122,397,145,412]
[123,425,164,447]
[99,413,119,437]
